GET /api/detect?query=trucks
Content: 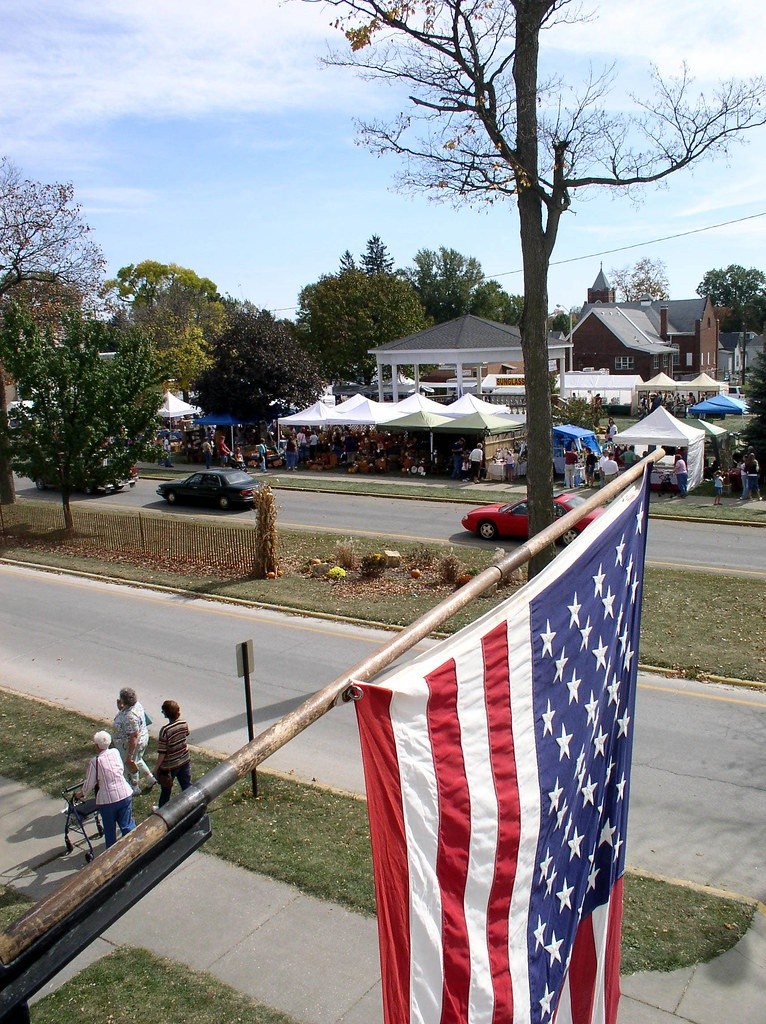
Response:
[28,429,140,496]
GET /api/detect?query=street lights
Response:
[555,303,574,371]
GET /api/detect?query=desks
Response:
[650,468,679,492]
[488,460,528,482]
[564,466,586,485]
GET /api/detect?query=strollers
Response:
[225,450,248,473]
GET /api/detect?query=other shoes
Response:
[714,503,722,505]
[758,497,762,501]
[148,777,157,789]
[132,789,141,796]
[679,494,688,498]
[152,806,159,812]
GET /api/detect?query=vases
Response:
[723,483,732,493]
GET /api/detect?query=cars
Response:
[460,492,609,548]
[728,385,746,401]
[155,467,267,512]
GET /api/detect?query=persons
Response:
[505,451,515,481]
[150,411,430,473]
[737,446,762,501]
[638,390,706,421]
[150,700,193,812]
[572,390,602,408]
[564,417,653,489]
[670,454,688,497]
[713,470,723,505]
[452,437,483,484]
[112,687,157,795]
[75,730,137,850]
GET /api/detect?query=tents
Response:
[154,390,203,417]
[635,372,729,421]
[688,394,750,434]
[554,374,644,416]
[278,392,527,461]
[612,404,706,496]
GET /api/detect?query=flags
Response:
[350,457,654,1024]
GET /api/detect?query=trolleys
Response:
[60,780,106,863]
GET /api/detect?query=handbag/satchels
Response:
[145,713,152,725]
[94,784,100,796]
